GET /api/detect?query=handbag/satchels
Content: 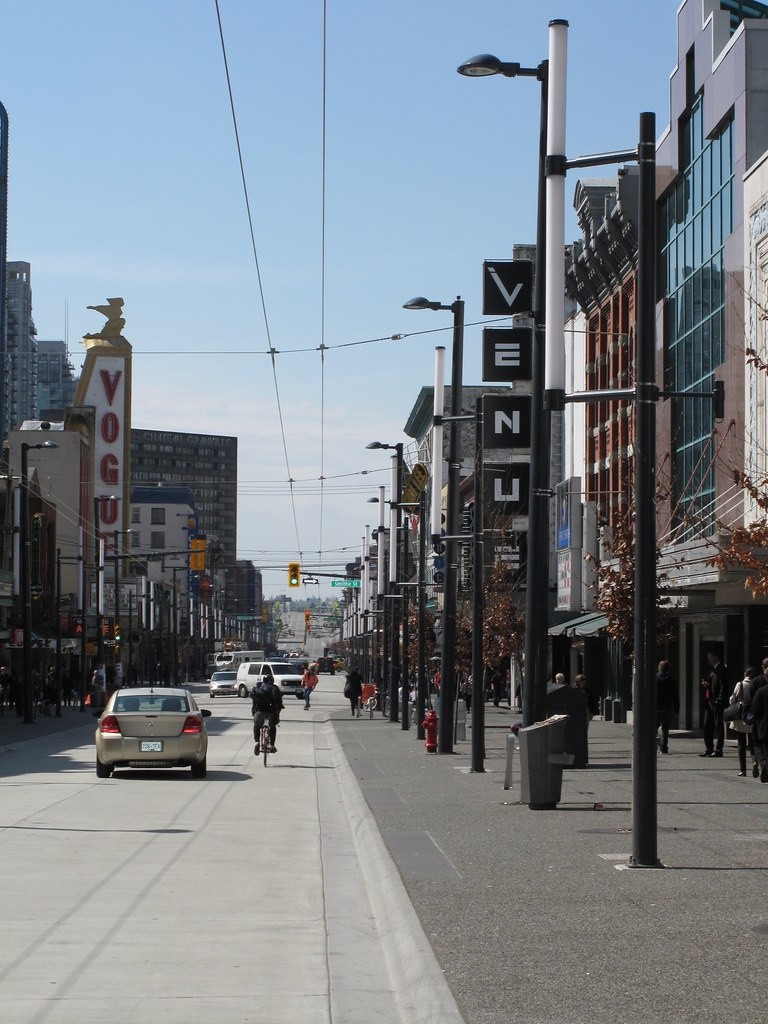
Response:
[723,700,743,722]
[344,687,350,698]
[295,684,305,699]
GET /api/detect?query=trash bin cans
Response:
[518,712,572,811]
[87,683,104,707]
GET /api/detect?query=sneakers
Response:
[254,742,260,756]
[270,746,277,752]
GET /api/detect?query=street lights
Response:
[366,442,403,722]
[405,298,465,754]
[20,439,60,722]
[93,497,136,705]
[457,54,552,724]
[55,554,84,718]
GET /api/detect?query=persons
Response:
[301,666,318,708]
[654,660,675,753]
[553,673,564,684]
[433,666,441,699]
[345,666,364,716]
[250,674,285,755]
[575,673,595,723]
[0,667,98,719]
[491,671,504,708]
[729,659,768,783]
[697,652,727,757]
[461,676,472,714]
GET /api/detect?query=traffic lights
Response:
[304,609,311,636]
[115,625,120,643]
[287,563,300,588]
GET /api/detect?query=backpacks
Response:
[249,687,274,713]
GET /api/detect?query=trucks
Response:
[216,650,266,671]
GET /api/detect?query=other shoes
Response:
[752,762,759,778]
[761,763,768,782]
[352,711,354,716]
[304,704,310,710]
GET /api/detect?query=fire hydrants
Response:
[420,707,440,756]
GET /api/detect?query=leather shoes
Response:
[698,749,723,758]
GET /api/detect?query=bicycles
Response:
[260,716,275,766]
[364,690,391,716]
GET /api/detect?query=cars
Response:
[266,648,310,670]
[206,672,235,697]
[314,648,348,676]
[94,686,213,779]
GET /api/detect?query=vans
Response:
[236,662,305,699]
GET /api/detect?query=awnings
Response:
[548,612,610,638]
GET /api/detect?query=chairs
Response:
[122,698,142,710]
[161,698,182,711]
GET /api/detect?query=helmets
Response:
[263,674,274,684]
